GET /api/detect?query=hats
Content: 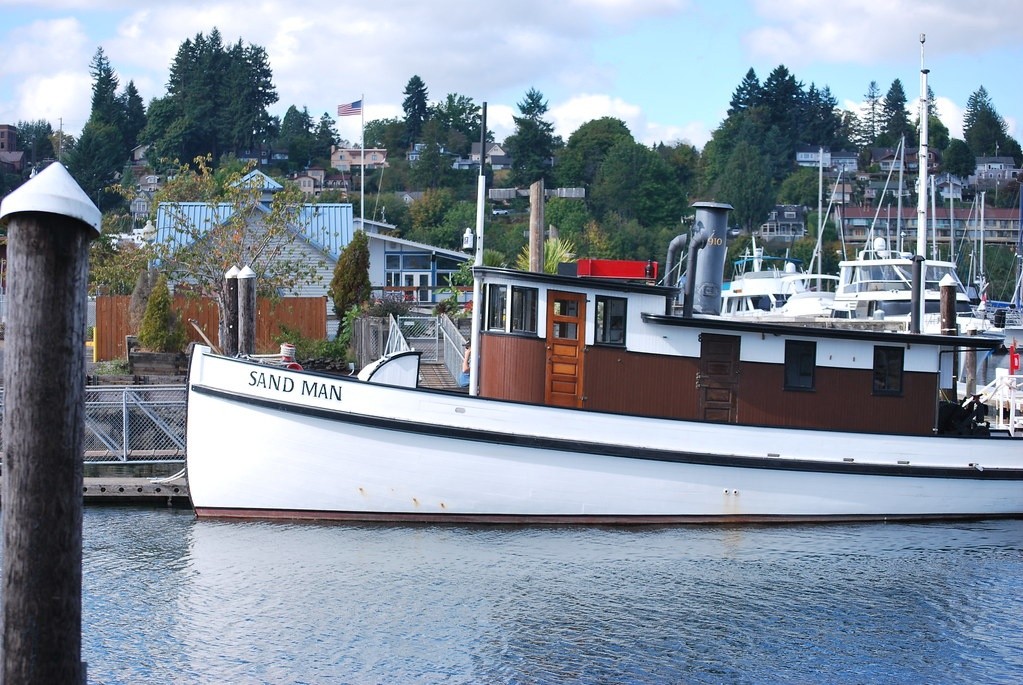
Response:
[462,341,471,346]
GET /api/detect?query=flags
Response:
[337,99,361,117]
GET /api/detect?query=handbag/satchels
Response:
[458,372,470,388]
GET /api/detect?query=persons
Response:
[459,338,471,388]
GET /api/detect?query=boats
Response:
[187,32,1023,528]
[720,134,1022,420]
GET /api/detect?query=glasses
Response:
[466,347,470,349]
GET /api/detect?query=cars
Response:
[492,208,508,215]
